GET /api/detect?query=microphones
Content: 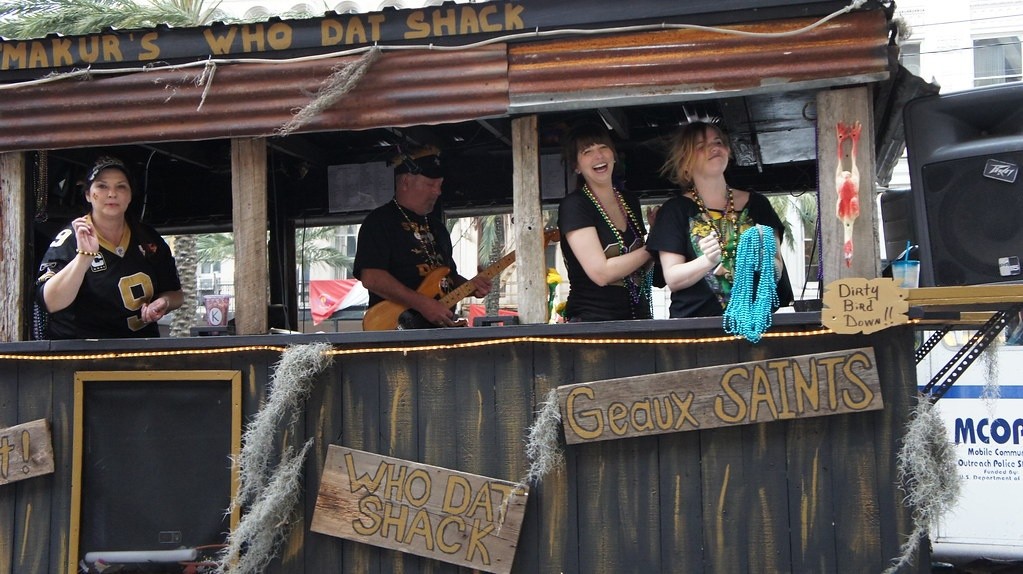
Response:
[400,154,424,175]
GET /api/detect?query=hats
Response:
[87,156,125,181]
[395,155,448,178]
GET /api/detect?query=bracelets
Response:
[75,249,99,256]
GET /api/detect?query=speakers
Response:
[903,80,1023,313]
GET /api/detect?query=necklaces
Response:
[723,224,780,344]
[692,183,738,283]
[583,182,654,318]
[391,197,451,297]
[815,120,824,280]
[33,151,48,224]
[34,300,48,340]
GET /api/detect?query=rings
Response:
[440,321,444,325]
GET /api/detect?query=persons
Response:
[645,120,783,319]
[33,155,184,340]
[353,145,493,330]
[556,121,661,322]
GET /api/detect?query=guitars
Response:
[360,222,562,333]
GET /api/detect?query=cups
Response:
[891,260,920,288]
[204,295,229,327]
